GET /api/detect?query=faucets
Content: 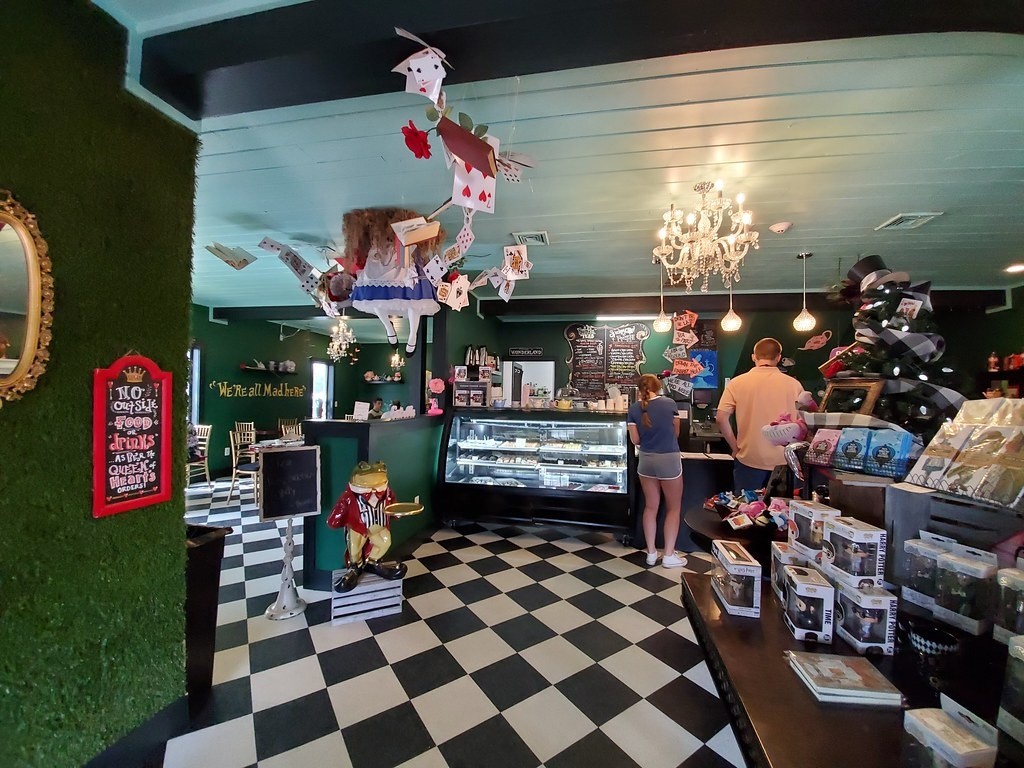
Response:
[702,415,711,429]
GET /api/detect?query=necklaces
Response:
[759,364,771,367]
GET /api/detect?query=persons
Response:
[715,521,869,631]
[368,397,384,420]
[850,602,883,639]
[390,400,401,410]
[628,374,688,570]
[715,338,806,497]
[342,207,441,358]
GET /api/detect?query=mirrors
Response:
[0,190,55,409]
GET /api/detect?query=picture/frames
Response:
[817,379,885,414]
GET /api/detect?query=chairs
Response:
[185,425,213,489]
[226,417,301,506]
[345,414,354,420]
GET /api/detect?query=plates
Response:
[456,437,627,471]
[573,407,586,410]
[382,501,424,516]
[249,438,304,448]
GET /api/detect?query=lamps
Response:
[326,308,357,364]
[721,274,742,331]
[653,260,672,333]
[390,348,406,372]
[651,184,759,293]
[792,252,816,331]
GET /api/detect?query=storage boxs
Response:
[710,540,761,618]
[803,427,913,478]
[771,500,1024,768]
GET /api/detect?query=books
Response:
[788,651,903,708]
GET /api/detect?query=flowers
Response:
[426,379,445,399]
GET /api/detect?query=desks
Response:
[248,444,280,453]
[683,503,772,547]
[254,428,279,440]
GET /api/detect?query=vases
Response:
[428,397,439,410]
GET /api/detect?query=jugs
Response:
[554,399,573,409]
[489,398,507,408]
[530,399,545,408]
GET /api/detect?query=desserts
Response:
[458,438,625,468]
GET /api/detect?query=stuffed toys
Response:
[704,490,791,531]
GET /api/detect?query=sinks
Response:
[693,423,722,436]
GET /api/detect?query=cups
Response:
[597,396,623,412]
[576,402,584,407]
[511,401,520,409]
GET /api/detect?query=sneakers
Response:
[646,550,661,565]
[662,550,688,569]
